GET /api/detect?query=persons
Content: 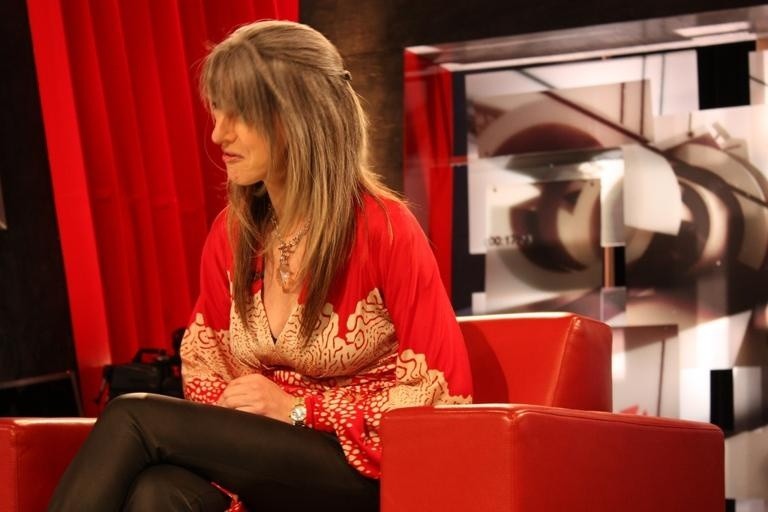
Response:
[45,19,476,512]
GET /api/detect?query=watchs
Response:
[288,394,306,428]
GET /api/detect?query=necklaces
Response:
[269,205,313,293]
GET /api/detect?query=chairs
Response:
[1,311,727,512]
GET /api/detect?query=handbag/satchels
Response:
[102,349,180,399]
